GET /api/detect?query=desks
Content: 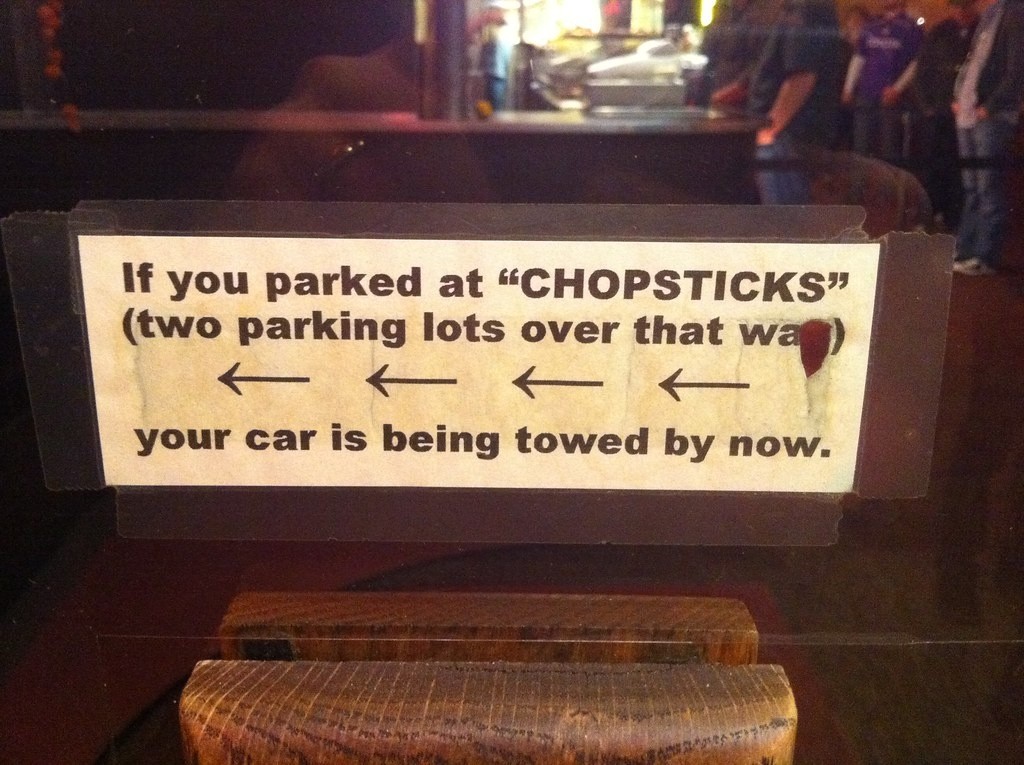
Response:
[0,101,773,210]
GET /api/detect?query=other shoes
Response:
[952,251,998,276]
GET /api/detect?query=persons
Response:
[464,0,518,115]
[21,1,91,136]
[676,0,1024,275]
